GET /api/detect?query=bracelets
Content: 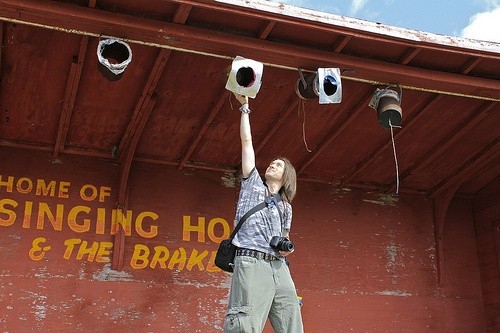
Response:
[239,107,251,114]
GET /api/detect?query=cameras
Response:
[269,236,294,252]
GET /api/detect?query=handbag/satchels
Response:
[215,239,236,273]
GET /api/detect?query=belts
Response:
[237,248,276,261]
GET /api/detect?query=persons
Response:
[223,92,304,333]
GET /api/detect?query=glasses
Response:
[265,197,274,210]
